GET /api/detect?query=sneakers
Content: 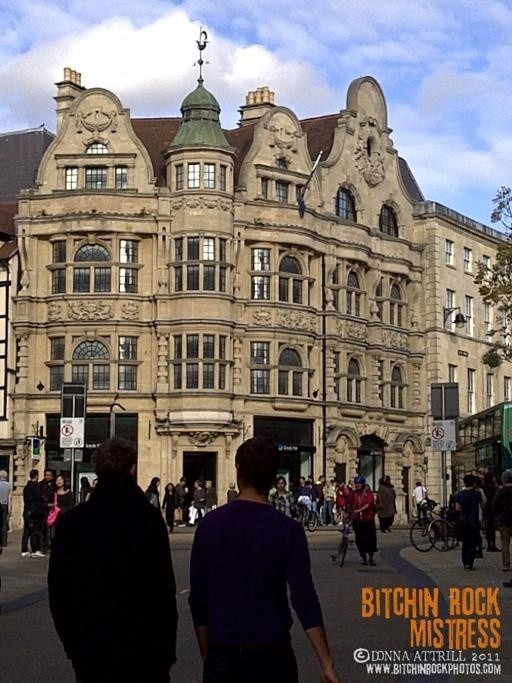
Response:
[30,550,45,557]
[361,560,367,564]
[21,551,31,557]
[369,558,376,564]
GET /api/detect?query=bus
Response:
[450,400,512,511]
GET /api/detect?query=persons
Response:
[453,468,511,587]
[48,436,179,682]
[187,436,341,682]
[411,481,431,526]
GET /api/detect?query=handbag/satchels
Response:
[45,505,61,526]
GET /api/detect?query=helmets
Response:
[355,476,365,482]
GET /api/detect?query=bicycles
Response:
[409,501,442,552]
[329,516,355,568]
[306,508,322,533]
[427,505,462,552]
